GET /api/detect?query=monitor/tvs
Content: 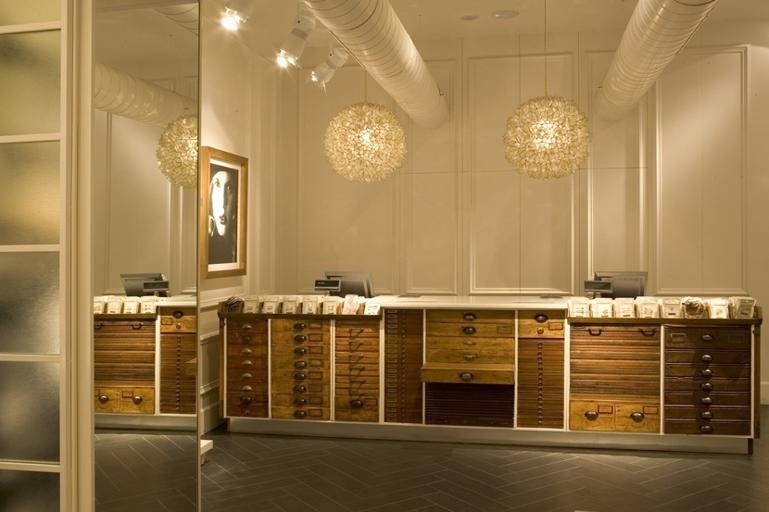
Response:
[120,273,170,296]
[323,272,374,298]
[594,271,647,297]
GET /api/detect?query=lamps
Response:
[219,0,253,32]
[156,114,200,189]
[323,67,408,182]
[275,12,316,66]
[311,47,348,85]
[504,1,592,180]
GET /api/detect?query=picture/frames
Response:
[199,146,249,280]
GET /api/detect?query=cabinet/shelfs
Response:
[224,318,269,418]
[566,324,662,436]
[516,310,567,432]
[269,318,333,421]
[662,324,754,438]
[92,295,199,432]
[332,320,384,423]
[420,309,516,385]
[379,309,425,425]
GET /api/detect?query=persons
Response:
[207,169,238,264]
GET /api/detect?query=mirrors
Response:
[91,0,200,512]
